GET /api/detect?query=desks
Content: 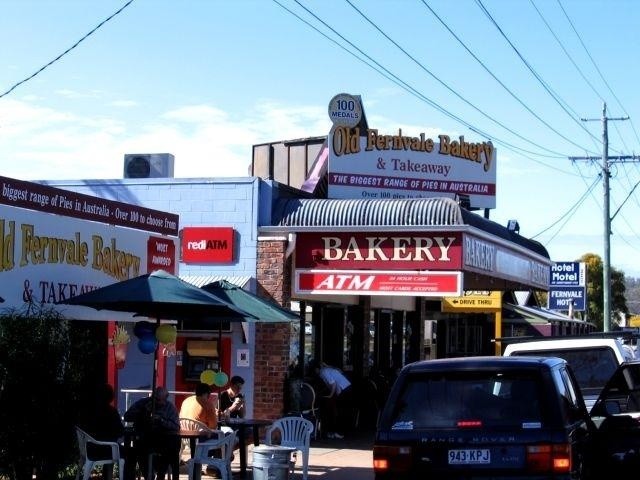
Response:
[217,416,277,480]
[173,429,215,480]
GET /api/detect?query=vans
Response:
[491,336,639,430]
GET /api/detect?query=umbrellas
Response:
[55,269,258,480]
[132,280,304,413]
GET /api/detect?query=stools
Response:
[136,451,171,480]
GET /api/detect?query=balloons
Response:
[199,370,228,388]
[133,321,177,354]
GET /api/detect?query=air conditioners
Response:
[122,153,175,179]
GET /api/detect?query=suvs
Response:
[372,353,639,477]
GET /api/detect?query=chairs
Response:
[177,418,211,474]
[74,425,126,480]
[266,416,314,480]
[354,378,382,432]
[288,378,321,443]
[187,428,240,480]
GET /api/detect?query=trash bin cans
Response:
[249,444,297,480]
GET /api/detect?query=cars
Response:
[621,344,639,361]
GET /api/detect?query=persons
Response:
[82,384,137,480]
[123,387,180,480]
[218,375,251,463]
[177,384,222,478]
[313,358,353,441]
